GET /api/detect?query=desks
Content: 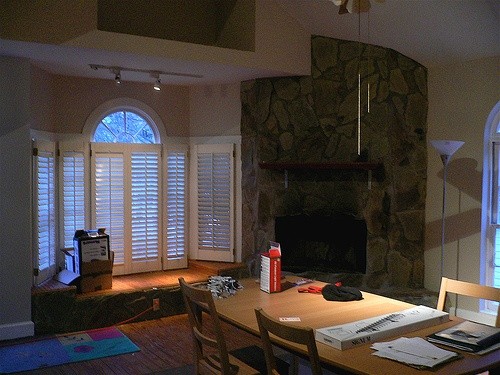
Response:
[190,276,500,375]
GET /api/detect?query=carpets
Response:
[0,326,141,375]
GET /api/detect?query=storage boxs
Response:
[260,241,282,293]
[54,227,116,294]
[315,306,451,352]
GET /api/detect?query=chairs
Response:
[177,277,322,375]
[434,277,500,328]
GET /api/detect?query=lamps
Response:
[428,139,465,279]
[149,71,161,91]
[111,69,121,85]
